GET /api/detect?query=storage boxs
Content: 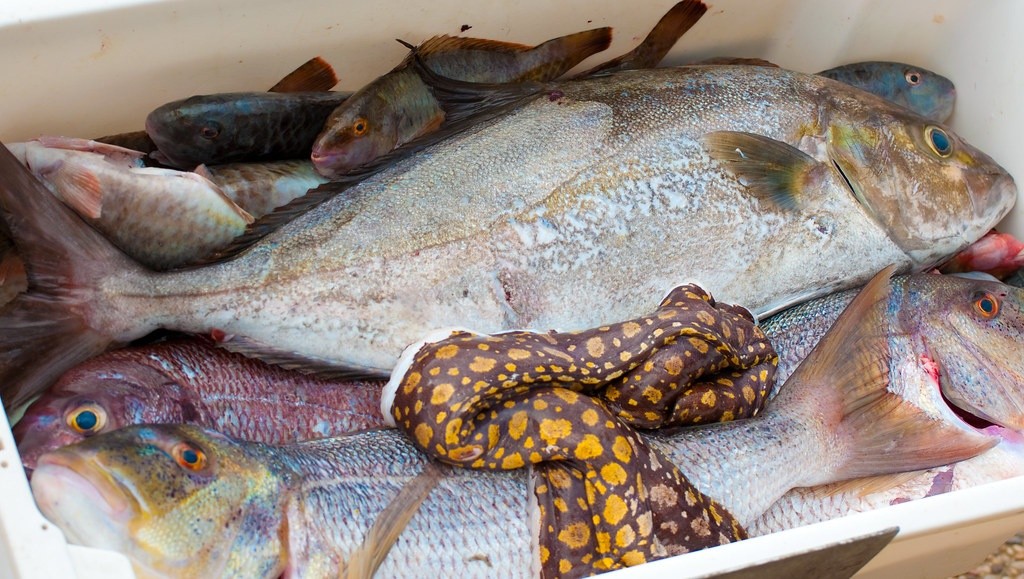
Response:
[0,0,1024,579]
